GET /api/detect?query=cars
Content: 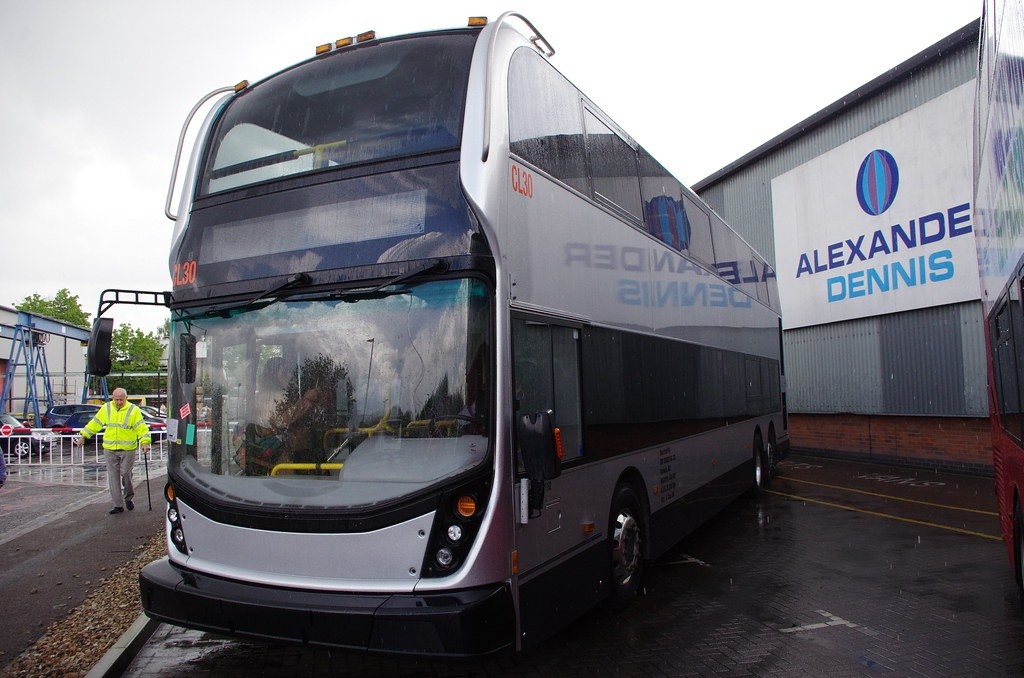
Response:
[0,414,58,458]
[60,409,168,446]
[138,404,167,418]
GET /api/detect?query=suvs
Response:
[42,404,104,444]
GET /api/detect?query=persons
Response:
[451,355,554,435]
[78,387,152,514]
[255,357,319,472]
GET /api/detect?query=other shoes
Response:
[109,506,124,514]
[125,499,134,510]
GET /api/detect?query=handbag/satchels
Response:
[252,435,284,474]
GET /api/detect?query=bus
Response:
[976,2,1024,592]
[87,10,793,667]
